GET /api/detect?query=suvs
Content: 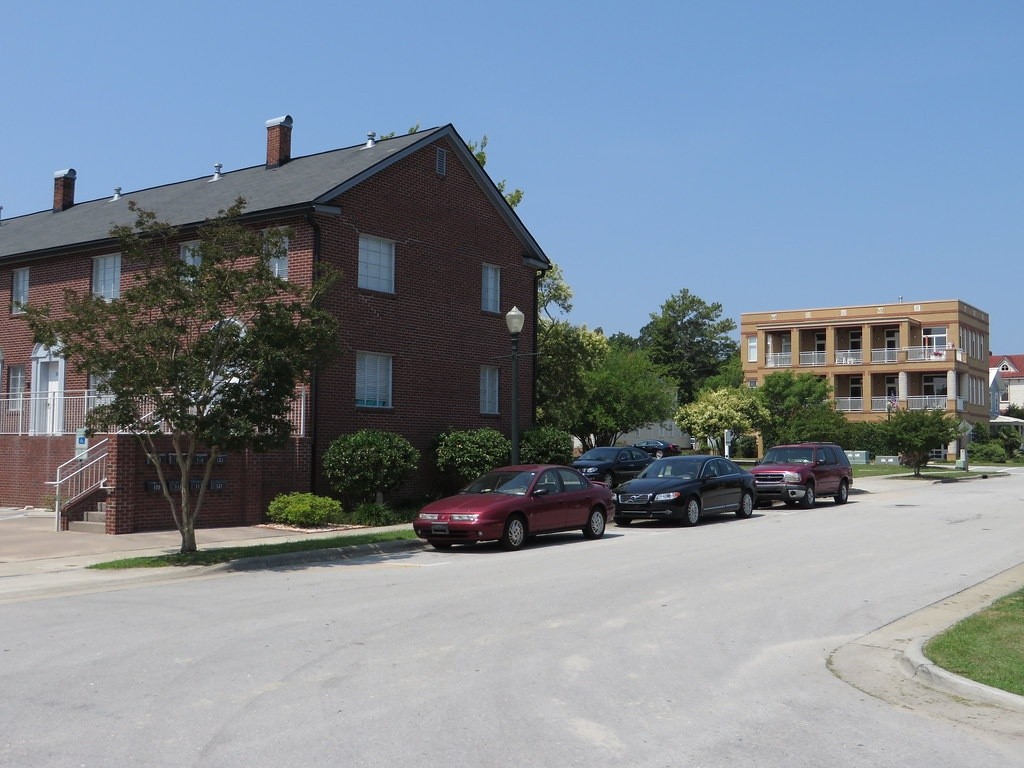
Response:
[746,441,853,510]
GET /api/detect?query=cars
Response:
[413,463,616,552]
[566,446,659,491]
[627,439,682,458]
[611,454,759,527]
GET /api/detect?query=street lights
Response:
[506,305,526,466]
[886,401,893,421]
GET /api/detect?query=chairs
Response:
[619,452,628,460]
